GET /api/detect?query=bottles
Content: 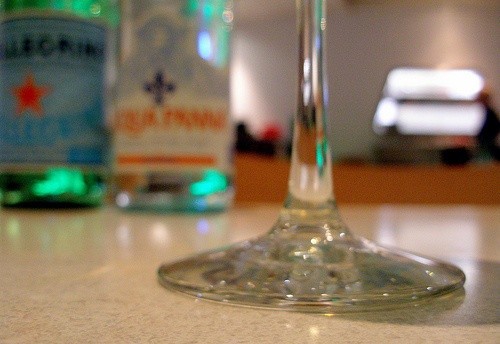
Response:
[112,0,236,213]
[0,0,121,211]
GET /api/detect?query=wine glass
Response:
[157,1,466,316]
